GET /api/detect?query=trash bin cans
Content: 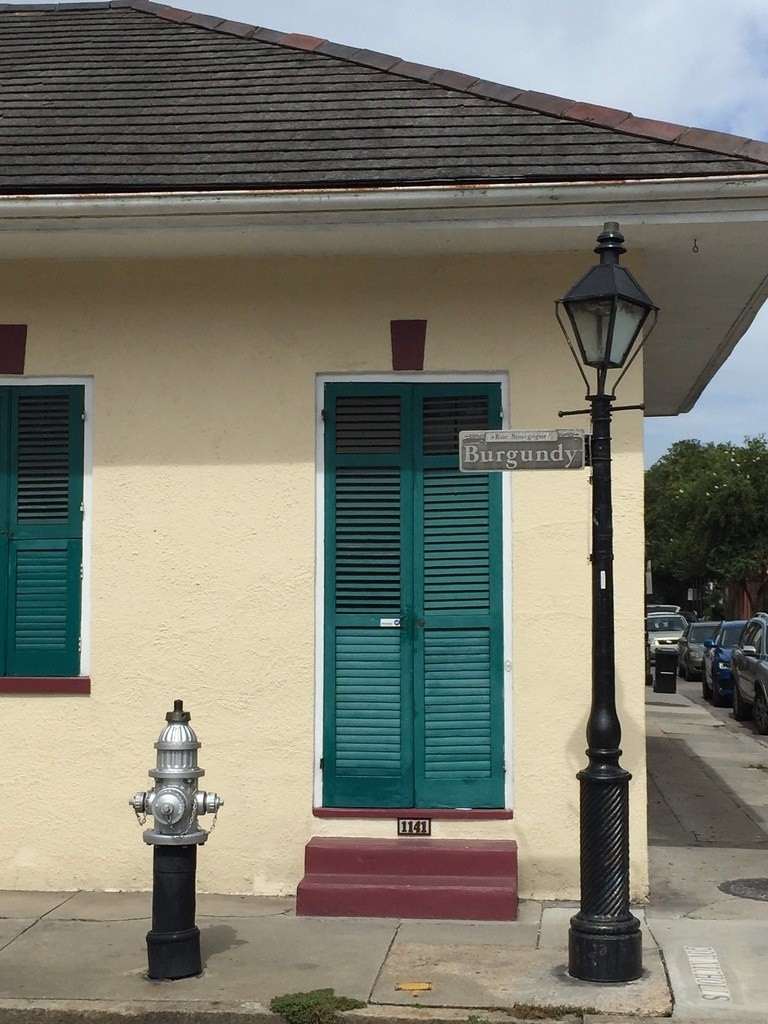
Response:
[653,647,679,694]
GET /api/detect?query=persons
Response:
[667,621,680,631]
[648,622,657,630]
[658,623,667,630]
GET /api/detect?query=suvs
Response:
[731,612,768,736]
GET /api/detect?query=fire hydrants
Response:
[129,700,224,982]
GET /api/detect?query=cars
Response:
[646,605,751,707]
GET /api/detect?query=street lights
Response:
[553,219,661,986]
